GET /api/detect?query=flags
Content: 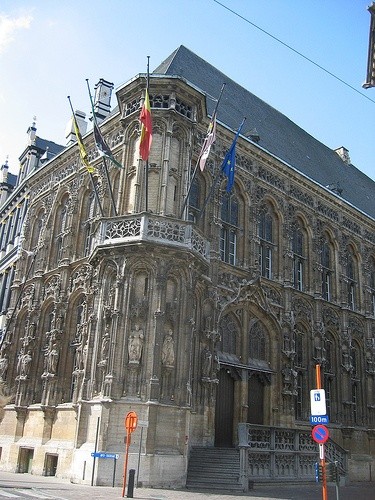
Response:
[139,87,152,162]
[90,94,123,168]
[74,117,93,173]
[220,122,244,193]
[199,114,217,172]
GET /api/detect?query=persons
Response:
[162,330,174,365]
[127,324,144,361]
[202,332,218,379]
[0,331,13,381]
[43,328,61,373]
[17,332,35,377]
[282,362,297,394]
